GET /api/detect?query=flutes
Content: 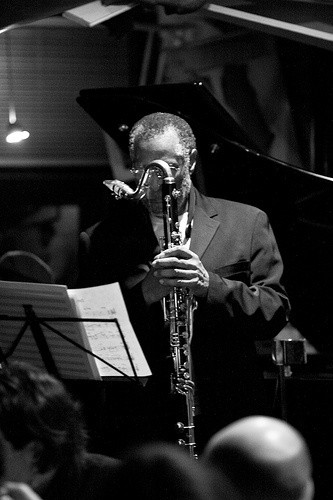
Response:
[99,159,203,463]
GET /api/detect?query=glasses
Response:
[129,158,185,180]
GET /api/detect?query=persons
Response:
[76,111,292,453]
[0,357,320,500]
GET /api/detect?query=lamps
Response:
[3,30,31,145]
